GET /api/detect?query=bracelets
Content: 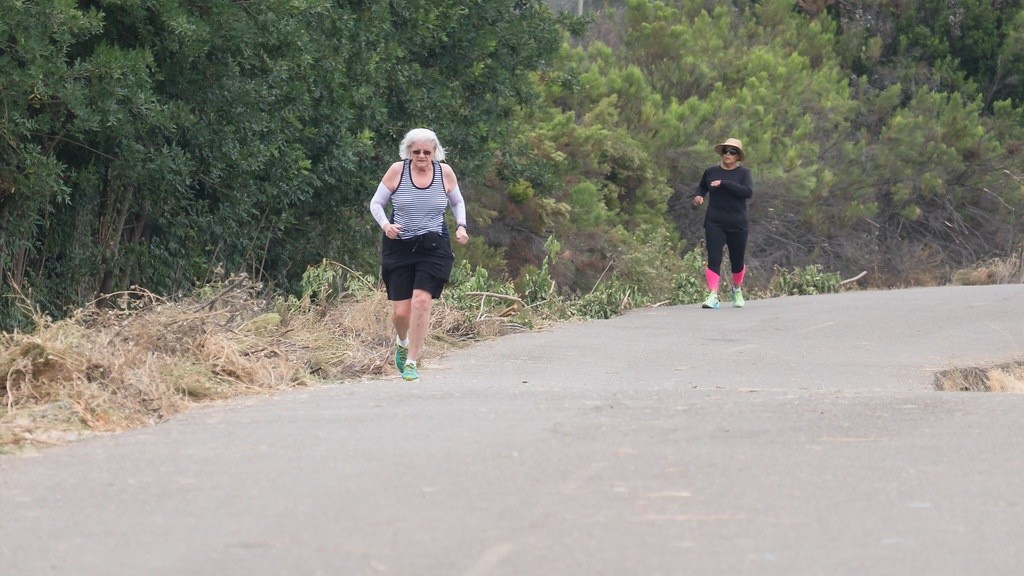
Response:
[456,224,468,231]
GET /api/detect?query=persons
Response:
[370,128,469,381]
[693,138,753,309]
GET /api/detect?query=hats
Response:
[714,138,744,161]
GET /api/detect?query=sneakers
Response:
[701,291,719,309]
[732,285,744,307]
[395,334,408,373]
[403,363,417,381]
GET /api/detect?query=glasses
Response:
[722,149,738,155]
[409,145,434,155]
